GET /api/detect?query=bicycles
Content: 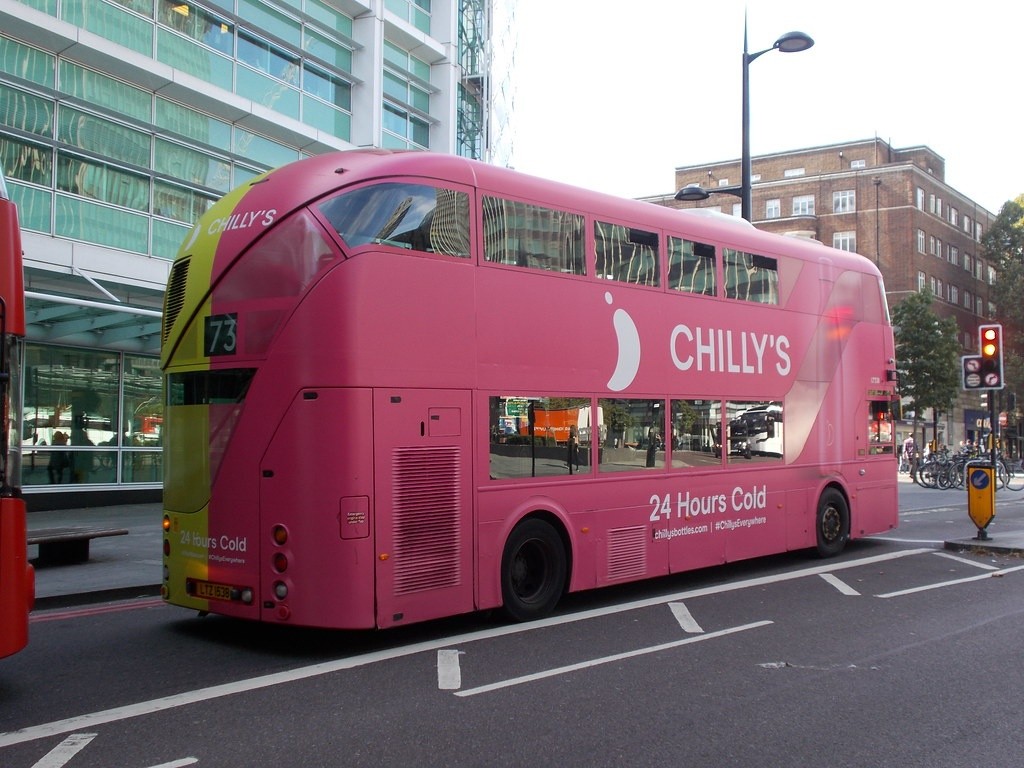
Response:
[909,438,1024,492]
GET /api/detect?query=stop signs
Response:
[998,412,1008,425]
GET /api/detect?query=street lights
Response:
[675,11,815,221]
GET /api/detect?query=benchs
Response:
[26,528,128,571]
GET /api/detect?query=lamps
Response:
[839,151,843,158]
[708,170,712,176]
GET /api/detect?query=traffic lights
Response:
[961,324,1004,390]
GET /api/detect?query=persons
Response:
[903,433,986,483]
[47,431,68,485]
[655,433,684,452]
[614,437,618,449]
[109,428,128,465]
[565,425,580,472]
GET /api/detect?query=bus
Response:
[157,147,914,626]
[0,150,42,663]
[11,401,163,450]
